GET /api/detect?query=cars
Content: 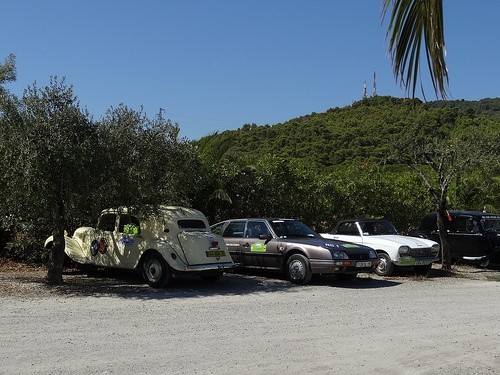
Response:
[413,209,500,270]
[319,218,441,276]
[43,202,239,287]
[212,217,379,284]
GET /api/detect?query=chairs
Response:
[228,227,244,237]
[364,224,375,235]
[247,227,261,238]
